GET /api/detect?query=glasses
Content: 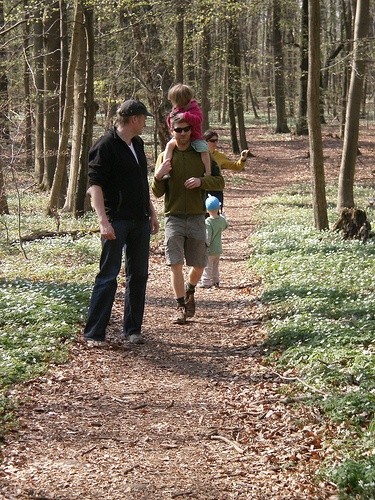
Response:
[173,125,191,133]
[207,139,219,143]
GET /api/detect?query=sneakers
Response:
[127,333,144,344]
[172,305,186,324]
[185,285,195,317]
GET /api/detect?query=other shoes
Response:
[210,285,219,289]
[199,284,209,289]
[84,337,108,346]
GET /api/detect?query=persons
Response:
[163,84,211,179]
[198,196,229,288]
[83,100,159,348]
[203,129,249,219]
[151,113,225,324]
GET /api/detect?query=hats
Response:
[205,196,220,209]
[119,99,154,117]
